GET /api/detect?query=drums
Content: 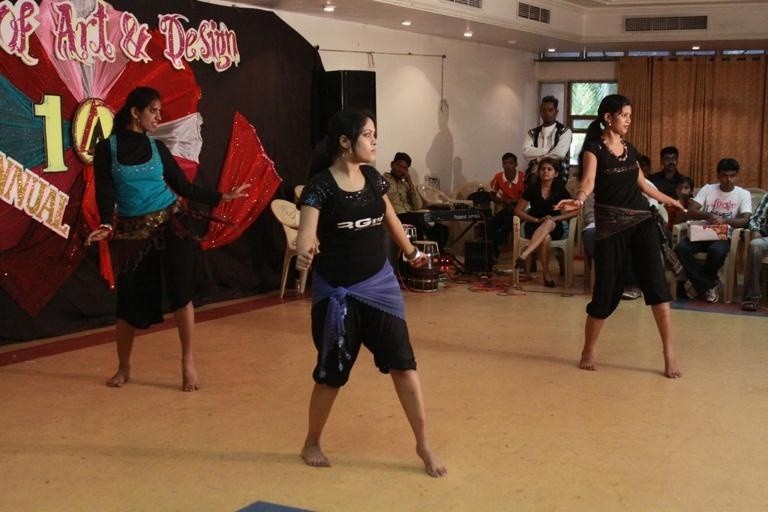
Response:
[401,223,417,242]
[400,239,440,293]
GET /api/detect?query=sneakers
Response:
[685,281,697,298]
[705,282,723,303]
[741,297,760,311]
[623,288,642,298]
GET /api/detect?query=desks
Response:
[409,207,492,274]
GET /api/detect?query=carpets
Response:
[236,500,311,512]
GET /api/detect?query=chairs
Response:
[456,182,501,215]
[741,229,768,300]
[271,199,320,299]
[513,205,582,288]
[670,187,766,303]
[417,184,474,210]
[294,185,306,202]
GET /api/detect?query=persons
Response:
[512,156,573,288]
[486,152,531,246]
[553,94,689,380]
[647,146,688,199]
[82,84,252,392]
[674,157,752,305]
[738,191,768,311]
[293,105,449,479]
[381,151,450,253]
[663,177,694,231]
[522,95,573,188]
[581,176,669,299]
[639,155,651,178]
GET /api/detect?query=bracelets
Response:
[534,218,538,223]
[98,223,113,231]
[402,246,421,263]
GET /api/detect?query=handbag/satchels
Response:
[687,219,731,242]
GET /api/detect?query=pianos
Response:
[397,203,493,223]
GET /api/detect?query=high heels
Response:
[514,257,525,270]
[542,276,555,287]
